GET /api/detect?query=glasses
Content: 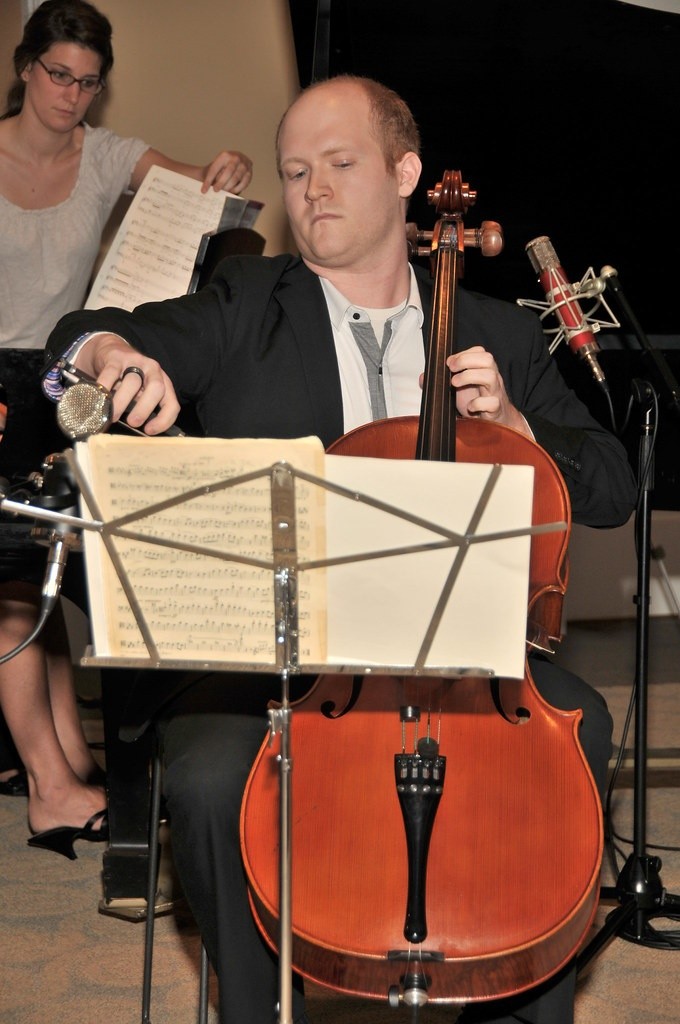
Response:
[36,58,106,95]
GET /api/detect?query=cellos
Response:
[236,170,605,1006]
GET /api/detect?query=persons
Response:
[39,70,645,1023]
[0,0,261,859]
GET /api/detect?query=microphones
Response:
[40,452,80,614]
[55,383,149,440]
[526,234,609,394]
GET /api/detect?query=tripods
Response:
[571,265,680,982]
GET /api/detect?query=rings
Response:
[121,366,145,386]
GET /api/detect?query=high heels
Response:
[28,808,109,861]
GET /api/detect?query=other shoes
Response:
[0,773,28,796]
